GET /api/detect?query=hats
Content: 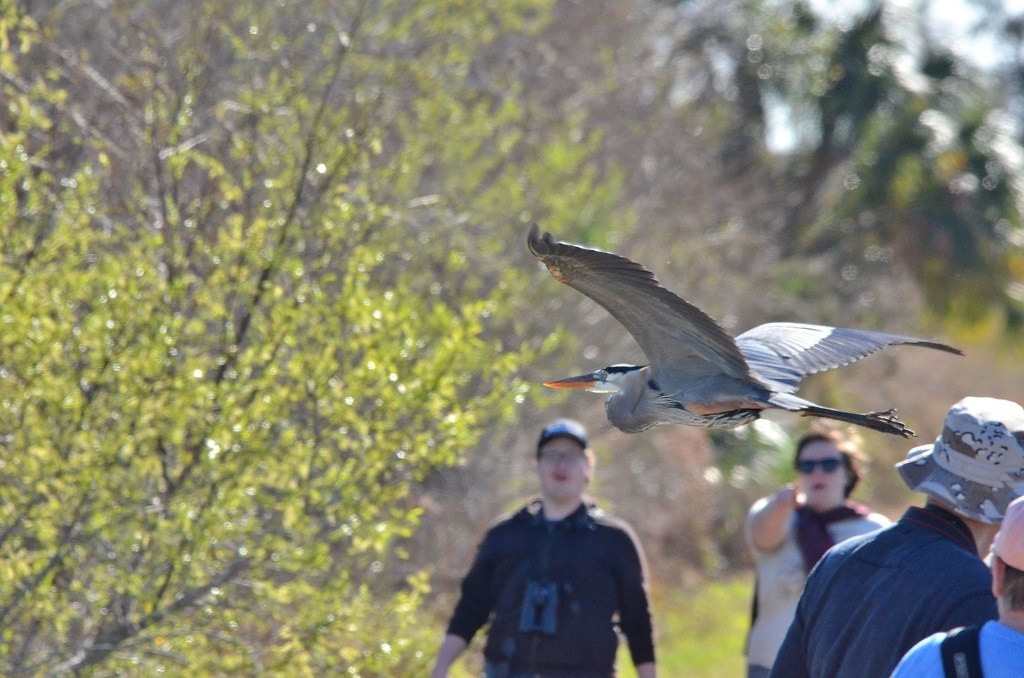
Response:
[536,419,588,450]
[991,496,1024,571]
[892,396,1024,524]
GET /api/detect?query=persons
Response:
[890,498,1022,678]
[742,427,893,678]
[767,395,1023,678]
[427,417,658,677]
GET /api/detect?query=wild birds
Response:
[527,224,969,438]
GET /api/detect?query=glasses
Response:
[539,449,586,468]
[794,455,845,475]
